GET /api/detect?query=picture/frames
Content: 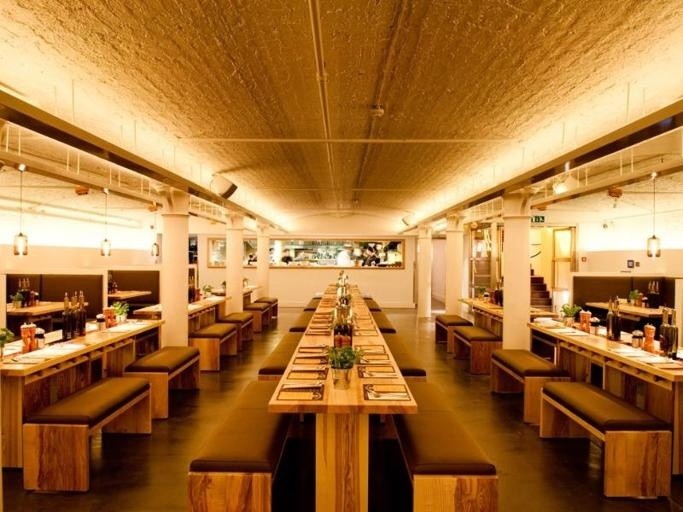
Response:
[207,236,405,269]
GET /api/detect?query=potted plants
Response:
[629,289,641,306]
[476,285,486,298]
[202,285,215,298]
[561,304,581,324]
[110,302,129,324]
[324,346,366,389]
[0,328,14,362]
[10,292,24,307]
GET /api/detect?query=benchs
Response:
[218,312,254,351]
[381,333,426,376]
[289,311,315,332]
[452,326,502,376]
[370,311,396,334]
[390,377,499,512]
[490,349,571,424]
[435,314,473,354]
[363,298,381,312]
[539,382,672,498]
[243,303,271,333]
[304,297,322,311]
[188,381,298,512]
[253,297,278,322]
[125,346,201,418]
[258,332,305,380]
[23,377,153,491]
[188,323,238,371]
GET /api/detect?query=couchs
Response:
[573,275,683,347]
[0,274,109,330]
[106,270,161,318]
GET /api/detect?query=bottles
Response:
[96,315,105,332]
[647,280,652,308]
[78,290,86,337]
[333,306,342,348]
[655,280,660,308]
[589,318,599,336]
[16,277,39,308]
[35,330,45,351]
[187,277,196,305]
[69,292,79,338]
[106,278,113,296]
[493,279,504,308]
[649,281,657,310]
[659,309,669,357]
[605,300,615,340]
[666,310,678,360]
[61,293,71,340]
[612,301,621,342]
[340,309,351,351]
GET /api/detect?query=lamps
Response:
[391,215,411,233]
[150,208,159,256]
[13,171,29,255]
[214,175,298,234]
[101,190,112,257]
[646,176,662,258]
